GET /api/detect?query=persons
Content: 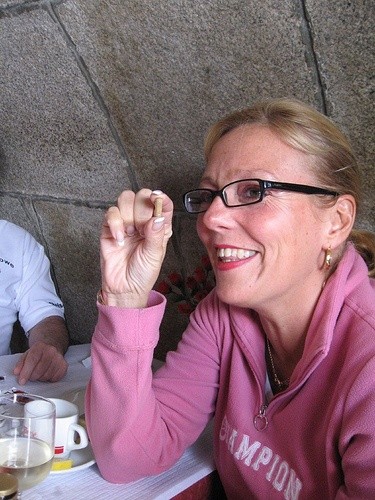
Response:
[0,219,70,385]
[84,94,375,500]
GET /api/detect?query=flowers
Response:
[155,256,216,315]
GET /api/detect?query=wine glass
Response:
[0,393,56,500]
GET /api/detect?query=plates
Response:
[49,443,96,474]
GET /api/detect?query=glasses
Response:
[182,178,339,216]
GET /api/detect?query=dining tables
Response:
[0,340,227,500]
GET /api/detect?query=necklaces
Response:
[266,333,289,391]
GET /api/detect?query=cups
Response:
[23,398,89,461]
